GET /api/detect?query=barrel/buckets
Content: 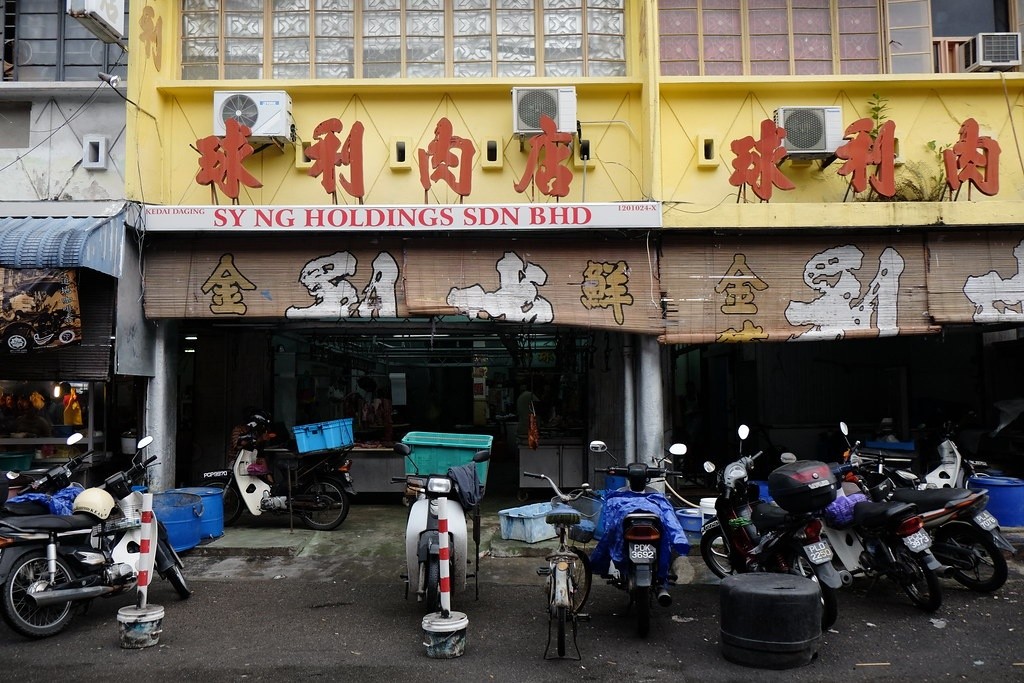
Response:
[967,476,1024,527]
[699,498,730,544]
[151,486,225,554]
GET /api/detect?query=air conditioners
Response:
[772,105,844,160]
[212,90,295,147]
[960,31,1021,71]
[509,86,578,141]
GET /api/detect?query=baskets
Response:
[551,494,603,543]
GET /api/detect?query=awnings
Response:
[0,207,126,278]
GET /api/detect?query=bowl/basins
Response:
[0,452,35,471]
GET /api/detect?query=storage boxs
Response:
[770,461,837,515]
[497,500,572,543]
[293,418,354,453]
[401,430,493,499]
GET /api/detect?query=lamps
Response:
[97,72,157,121]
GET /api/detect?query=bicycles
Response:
[524,471,606,660]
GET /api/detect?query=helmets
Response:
[72,488,116,520]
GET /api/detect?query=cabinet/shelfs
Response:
[516,438,587,488]
[340,447,407,495]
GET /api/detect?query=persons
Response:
[52,383,83,425]
[683,381,704,442]
[227,405,259,463]
[517,381,540,434]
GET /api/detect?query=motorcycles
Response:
[389,442,490,612]
[0,431,193,637]
[700,424,1016,631]
[197,414,355,531]
[589,439,687,637]
